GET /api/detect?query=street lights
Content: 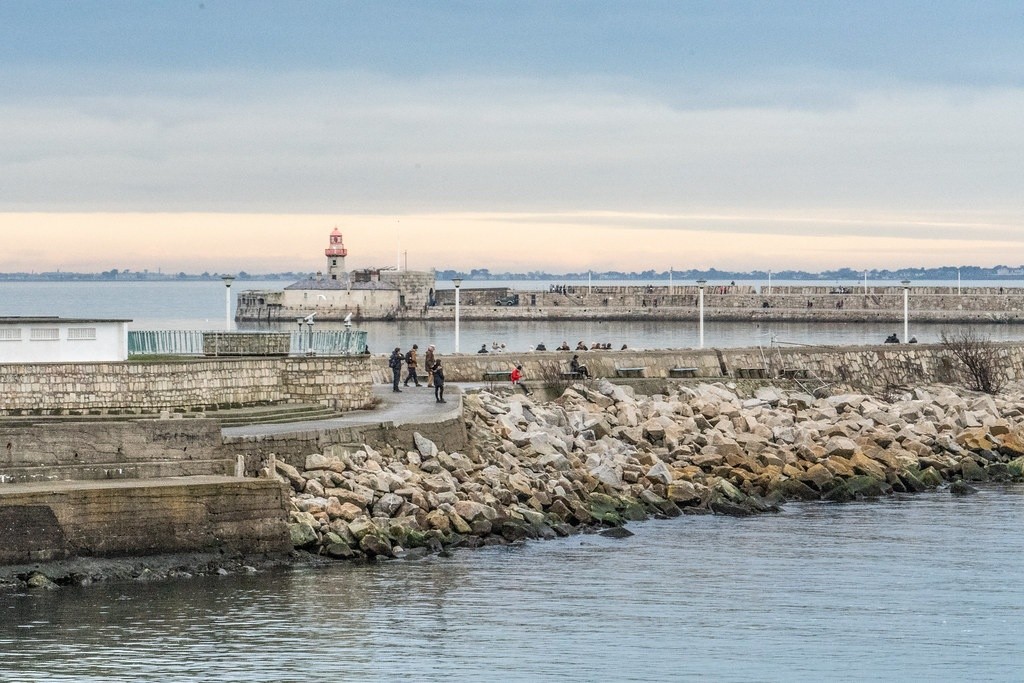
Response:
[695,276,708,349]
[900,277,911,343]
[451,275,464,354]
[222,274,235,331]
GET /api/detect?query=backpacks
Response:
[389,355,398,368]
[405,351,414,364]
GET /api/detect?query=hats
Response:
[413,345,418,349]
[517,365,522,371]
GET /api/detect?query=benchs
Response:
[484,372,513,380]
[783,369,809,379]
[562,372,582,379]
[737,368,765,378]
[670,368,698,376]
[619,368,644,378]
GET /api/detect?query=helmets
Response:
[428,346,435,352]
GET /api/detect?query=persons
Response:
[646,284,653,293]
[425,345,435,387]
[570,355,593,379]
[404,344,422,387]
[884,333,918,343]
[641,298,647,307]
[491,341,509,352]
[432,359,447,404]
[536,341,627,350]
[477,344,488,353]
[389,347,405,392]
[512,366,533,396]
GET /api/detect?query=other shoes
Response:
[440,399,446,404]
[416,383,421,387]
[530,392,534,395]
[525,392,530,398]
[393,389,402,393]
[404,384,409,387]
[428,384,434,388]
[436,399,440,404]
